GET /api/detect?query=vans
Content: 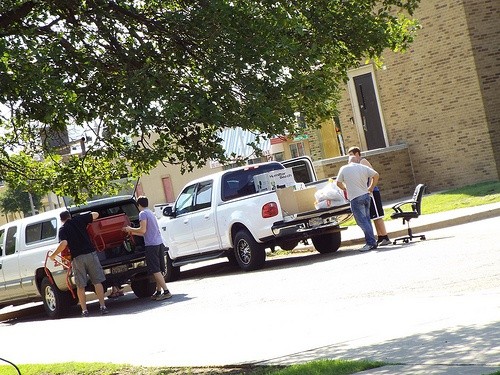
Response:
[0,194,156,317]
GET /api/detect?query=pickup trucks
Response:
[158,156,370,284]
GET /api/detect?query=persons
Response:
[336,155,380,252]
[120,195,172,301]
[49,211,110,316]
[348,146,391,246]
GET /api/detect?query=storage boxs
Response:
[251,166,320,217]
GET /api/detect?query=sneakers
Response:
[99,308,109,314]
[359,245,377,251]
[152,294,161,299]
[156,292,171,300]
[379,239,391,246]
[81,311,89,316]
[107,291,124,298]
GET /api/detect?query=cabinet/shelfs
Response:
[87,213,137,251]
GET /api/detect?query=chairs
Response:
[389,183,427,245]
